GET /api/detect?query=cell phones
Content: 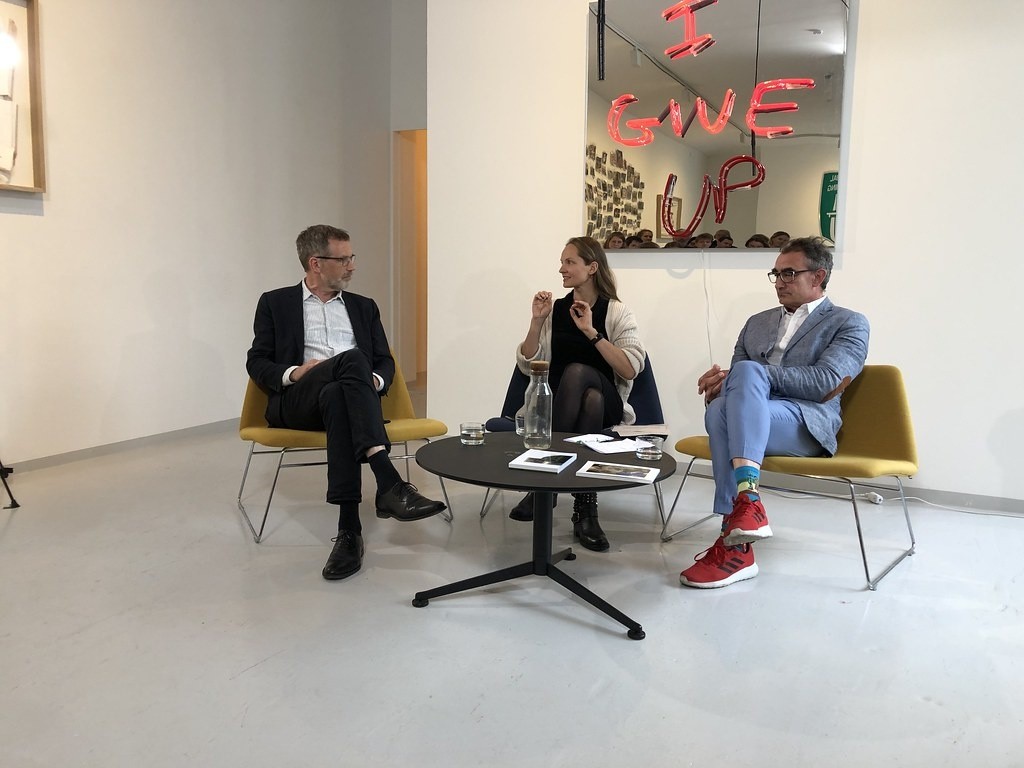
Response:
[506,415,516,421]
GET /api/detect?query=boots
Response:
[571,491,609,551]
[509,491,558,521]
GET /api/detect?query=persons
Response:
[246,224,448,581]
[508,236,646,552]
[603,229,660,249]
[664,229,738,249]
[745,231,790,248]
[679,236,870,590]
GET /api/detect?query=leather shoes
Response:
[322,530,364,580]
[375,481,447,522]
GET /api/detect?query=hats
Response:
[744,233,770,248]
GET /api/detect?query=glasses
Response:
[308,253,356,267]
[768,270,813,283]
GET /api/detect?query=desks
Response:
[412,432,676,640]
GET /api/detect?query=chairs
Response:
[479,351,667,526]
[660,366,919,591]
[237,346,454,543]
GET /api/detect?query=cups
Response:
[635,435,664,460]
[515,415,538,436]
[460,421,486,445]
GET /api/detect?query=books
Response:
[508,449,577,474]
[576,461,660,484]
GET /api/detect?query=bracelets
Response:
[591,333,604,345]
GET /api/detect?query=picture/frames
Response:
[656,195,683,239]
[0,0,47,193]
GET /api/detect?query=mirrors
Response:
[581,1,859,253]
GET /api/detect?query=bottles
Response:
[523,361,553,450]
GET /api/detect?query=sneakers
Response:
[679,536,758,589]
[723,493,773,546]
